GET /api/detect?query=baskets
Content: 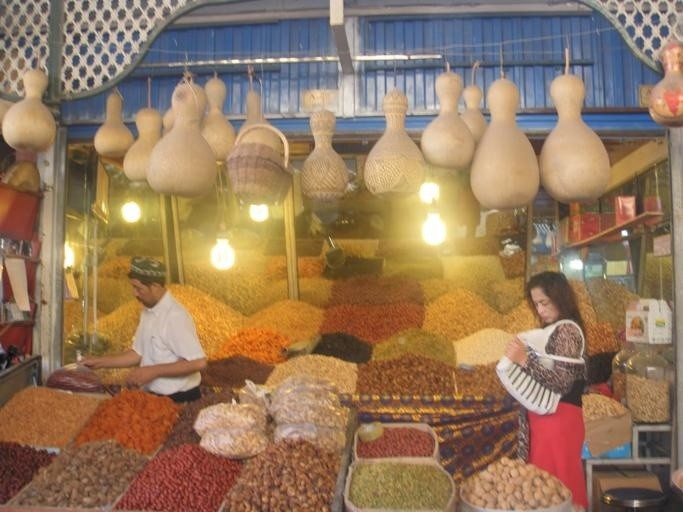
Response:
[226,124,293,204]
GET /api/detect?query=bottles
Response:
[29,232,41,260]
[611,340,674,425]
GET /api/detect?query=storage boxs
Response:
[592,470,665,512]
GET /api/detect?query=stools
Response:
[599,487,667,512]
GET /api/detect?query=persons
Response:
[503,272,588,510]
[77,256,208,403]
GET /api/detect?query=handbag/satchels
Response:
[495,324,561,416]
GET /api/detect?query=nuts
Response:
[0,351,671,512]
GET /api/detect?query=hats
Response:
[130,255,167,277]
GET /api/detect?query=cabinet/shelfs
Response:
[584,422,678,512]
